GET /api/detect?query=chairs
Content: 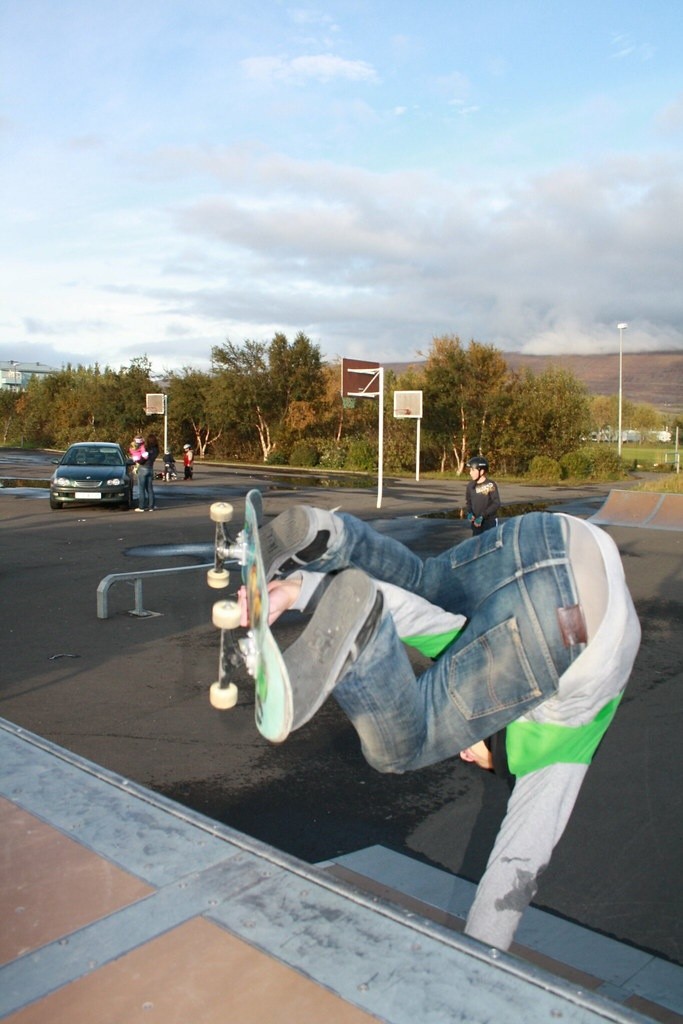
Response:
[103,454,117,462]
[75,450,87,460]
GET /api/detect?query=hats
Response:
[134,434,143,443]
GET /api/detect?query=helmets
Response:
[466,457,489,468]
[183,444,192,449]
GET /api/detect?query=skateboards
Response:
[205,489,294,743]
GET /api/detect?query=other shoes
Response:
[132,470,138,476]
[181,478,186,480]
[135,508,144,512]
[171,473,176,476]
[187,478,193,480]
[149,509,153,511]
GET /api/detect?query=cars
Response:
[49,441,137,512]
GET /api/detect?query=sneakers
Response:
[258,505,342,581]
[281,570,384,733]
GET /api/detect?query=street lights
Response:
[616,322,628,459]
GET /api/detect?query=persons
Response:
[134,435,160,512]
[463,457,500,536]
[181,444,194,480]
[237,504,641,953]
[163,449,177,481]
[130,434,147,474]
